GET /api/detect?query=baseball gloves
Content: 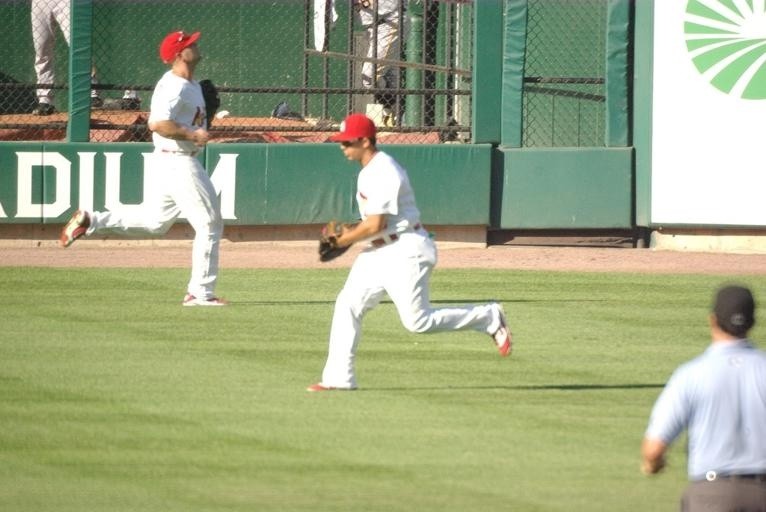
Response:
[319,221,352,261]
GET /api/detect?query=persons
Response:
[359,0,412,127]
[640,286,766,512]
[31,0,102,114]
[306,113,512,392]
[61,30,231,306]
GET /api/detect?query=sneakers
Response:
[306,384,352,391]
[493,303,511,354]
[183,294,228,306]
[32,104,54,115]
[90,97,102,109]
[62,211,90,247]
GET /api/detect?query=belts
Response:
[372,224,420,247]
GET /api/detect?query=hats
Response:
[324,114,376,142]
[717,287,754,332]
[160,31,200,62]
